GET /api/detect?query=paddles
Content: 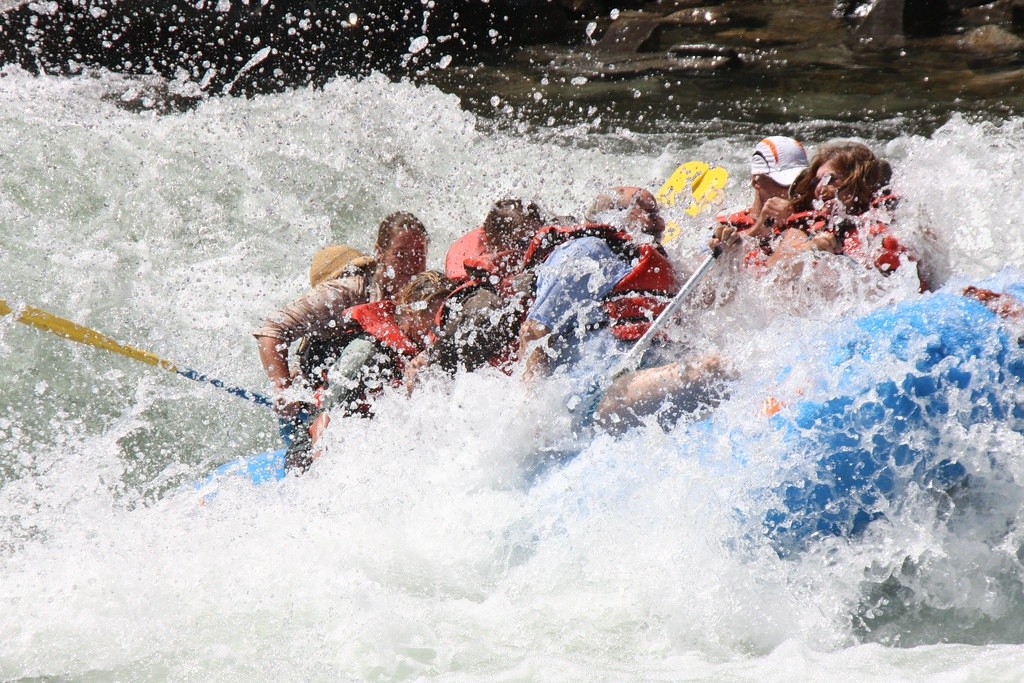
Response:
[609,235,740,371]
[642,160,730,244]
[1,298,281,410]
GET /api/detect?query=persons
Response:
[587,134,941,424]
[250,185,668,477]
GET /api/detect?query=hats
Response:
[751,135,812,187]
[308,244,377,289]
